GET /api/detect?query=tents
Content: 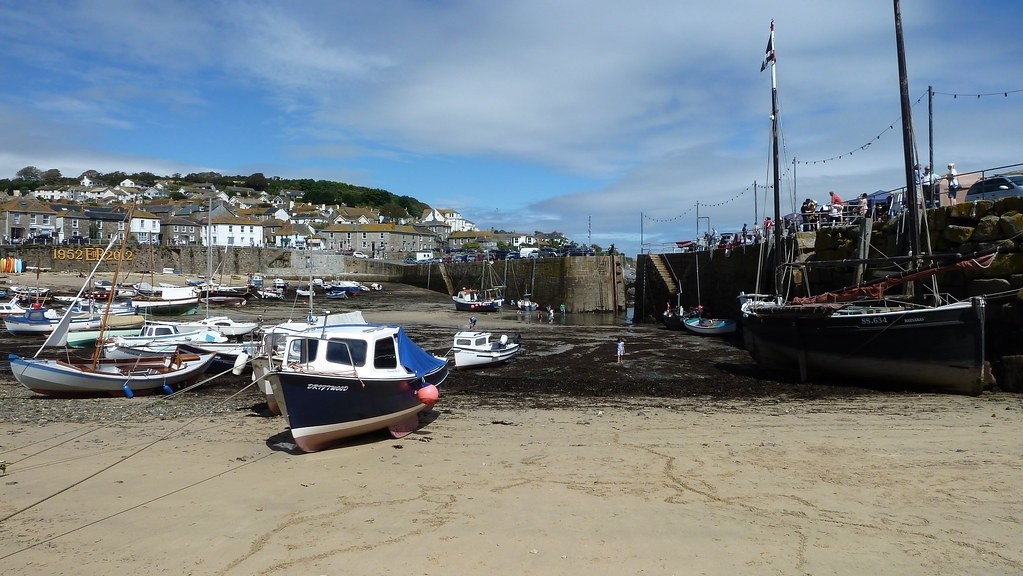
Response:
[848,190,893,204]
[783,212,803,225]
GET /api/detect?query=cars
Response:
[965,174,1023,202]
[30,234,53,243]
[139,239,158,246]
[62,236,84,245]
[404,247,595,264]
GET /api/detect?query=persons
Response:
[801,199,818,231]
[667,299,672,313]
[915,164,942,209]
[711,227,717,237]
[704,231,712,250]
[946,163,960,205]
[608,244,612,256]
[538,302,566,324]
[886,192,894,210]
[829,191,843,218]
[852,193,868,220]
[753,222,759,238]
[511,298,523,314]
[469,316,477,330]
[764,216,775,240]
[514,333,522,348]
[742,223,748,246]
[616,338,625,364]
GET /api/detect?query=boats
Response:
[659,294,699,330]
[683,250,736,335]
[517,283,538,310]
[264,309,449,454]
[454,331,520,371]
[452,263,507,311]
[0,193,383,416]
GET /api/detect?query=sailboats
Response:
[735,1,1023,398]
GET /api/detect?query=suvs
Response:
[353,252,369,259]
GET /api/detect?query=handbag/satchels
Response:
[953,182,963,192]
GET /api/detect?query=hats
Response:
[948,163,953,167]
